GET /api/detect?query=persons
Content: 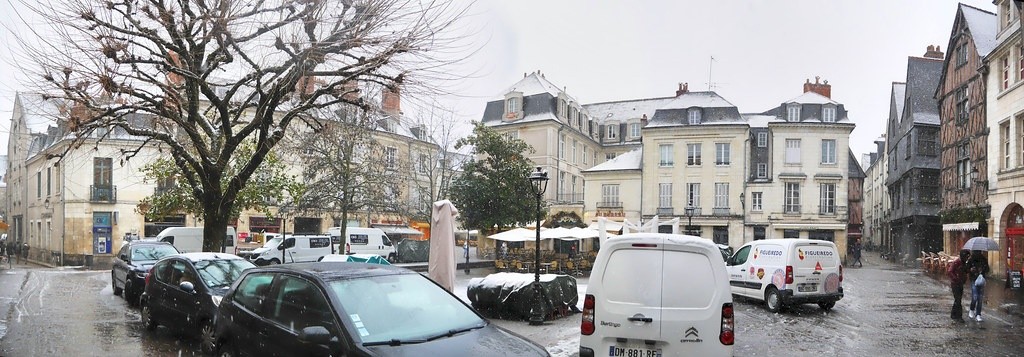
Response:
[0,238,30,264]
[966,250,989,321]
[947,249,970,323]
[850,239,863,268]
[463,241,467,259]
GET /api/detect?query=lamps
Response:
[971,166,989,186]
[740,192,744,209]
[44,199,60,210]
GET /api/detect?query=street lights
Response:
[527,166,550,325]
[685,202,697,235]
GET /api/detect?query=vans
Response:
[724,237,843,312]
[155,226,238,256]
[326,226,397,264]
[578,232,735,357]
[247,232,333,268]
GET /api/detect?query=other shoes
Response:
[969,308,974,319]
[951,311,956,319]
[957,316,964,323]
[976,314,983,322]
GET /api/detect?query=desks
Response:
[540,263,554,274]
[521,262,534,273]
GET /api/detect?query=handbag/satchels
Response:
[974,274,986,287]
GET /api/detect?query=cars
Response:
[139,251,274,357]
[211,261,552,357]
[111,238,186,308]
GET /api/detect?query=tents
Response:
[0,221,11,269]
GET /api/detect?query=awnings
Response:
[942,222,980,231]
[588,222,623,231]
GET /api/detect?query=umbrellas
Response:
[487,219,618,275]
[454,228,478,240]
[963,233,999,255]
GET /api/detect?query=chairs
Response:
[921,250,954,279]
[493,247,598,277]
[180,270,191,284]
